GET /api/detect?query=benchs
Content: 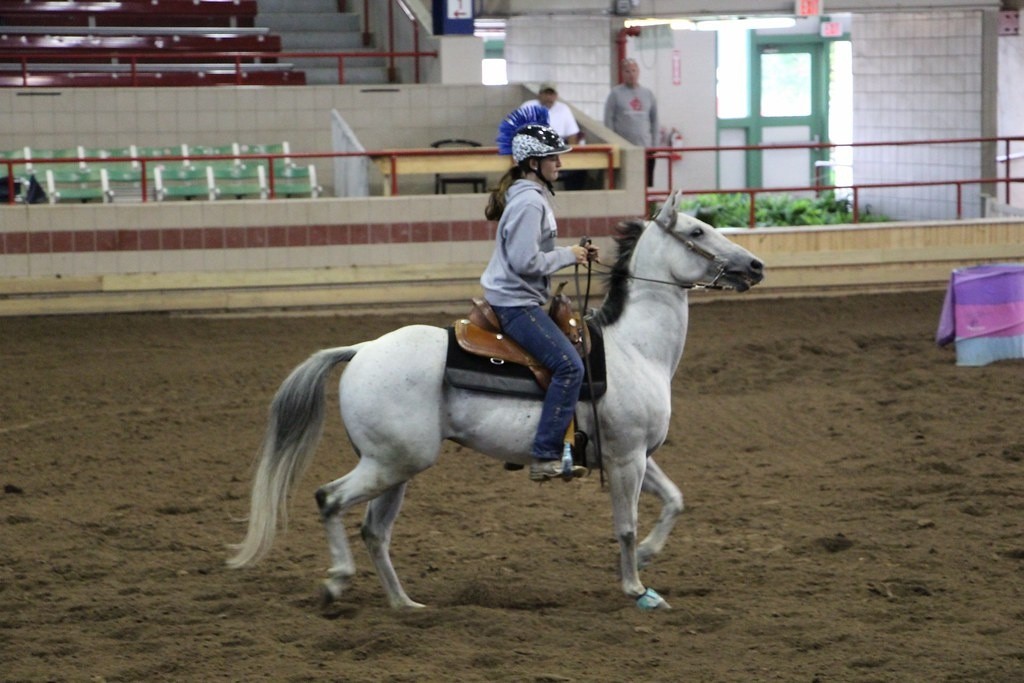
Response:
[1,141,320,203]
[0,0,307,85]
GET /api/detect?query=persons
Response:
[480,123,589,482]
[604,58,658,187]
[538,82,580,145]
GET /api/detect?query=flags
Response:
[331,110,368,197]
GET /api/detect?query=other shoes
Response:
[528,459,588,482]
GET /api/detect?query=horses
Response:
[226,192,766,612]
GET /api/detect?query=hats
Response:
[540,82,558,93]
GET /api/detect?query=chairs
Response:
[431,138,488,195]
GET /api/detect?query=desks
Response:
[372,142,622,196]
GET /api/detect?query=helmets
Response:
[497,105,572,163]
